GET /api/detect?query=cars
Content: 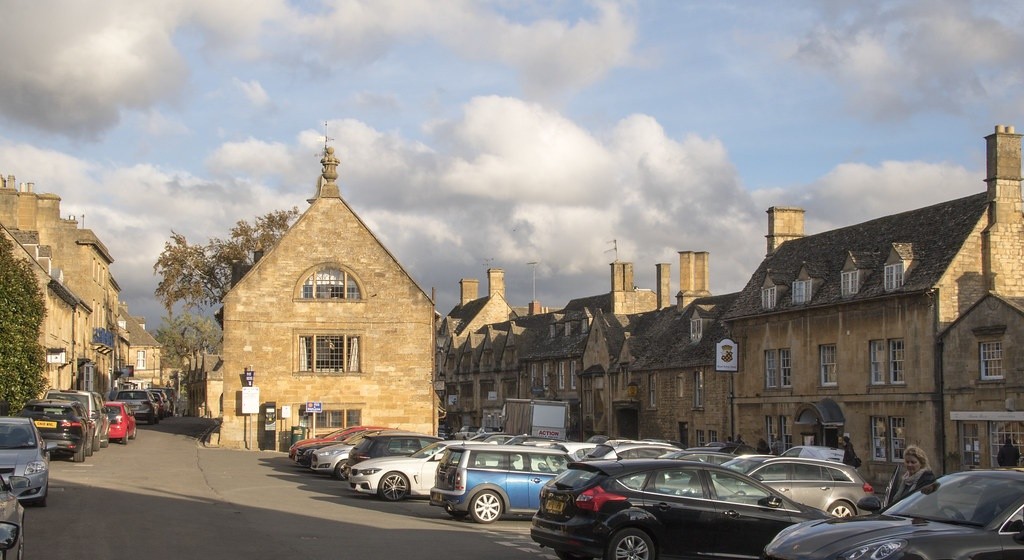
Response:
[530,456,841,560]
[288,426,882,504]
[0,389,138,560]
[113,387,187,425]
[429,443,578,524]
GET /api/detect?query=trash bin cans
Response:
[291,426,306,446]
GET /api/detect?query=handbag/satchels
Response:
[852,457,862,468]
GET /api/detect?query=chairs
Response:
[998,488,1023,510]
[8,428,29,443]
[410,443,420,451]
[656,474,666,492]
[538,463,551,471]
[510,456,516,469]
[687,476,703,497]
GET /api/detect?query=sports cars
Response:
[759,467,1024,560]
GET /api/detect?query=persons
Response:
[757,438,772,455]
[735,434,745,444]
[477,427,486,434]
[726,436,734,444]
[997,438,1020,468]
[921,482,940,495]
[768,432,785,456]
[892,444,936,503]
[109,387,119,401]
[499,426,503,433]
[103,387,112,401]
[843,435,858,467]
